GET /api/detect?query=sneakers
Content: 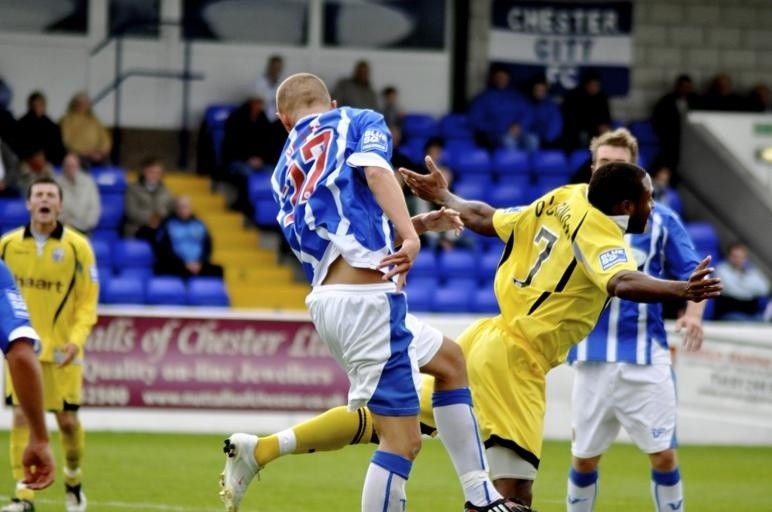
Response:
[64,481,88,512]
[0,497,34,511]
[464,496,537,512]
[218,432,264,512]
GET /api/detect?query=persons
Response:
[565,129,710,511]
[223,55,284,167]
[268,71,531,510]
[330,54,461,254]
[1,88,224,280]
[647,166,671,206]
[652,72,772,156]
[0,258,56,490]
[219,152,724,511]
[709,238,772,320]
[466,65,615,156]
[0,177,100,511]
